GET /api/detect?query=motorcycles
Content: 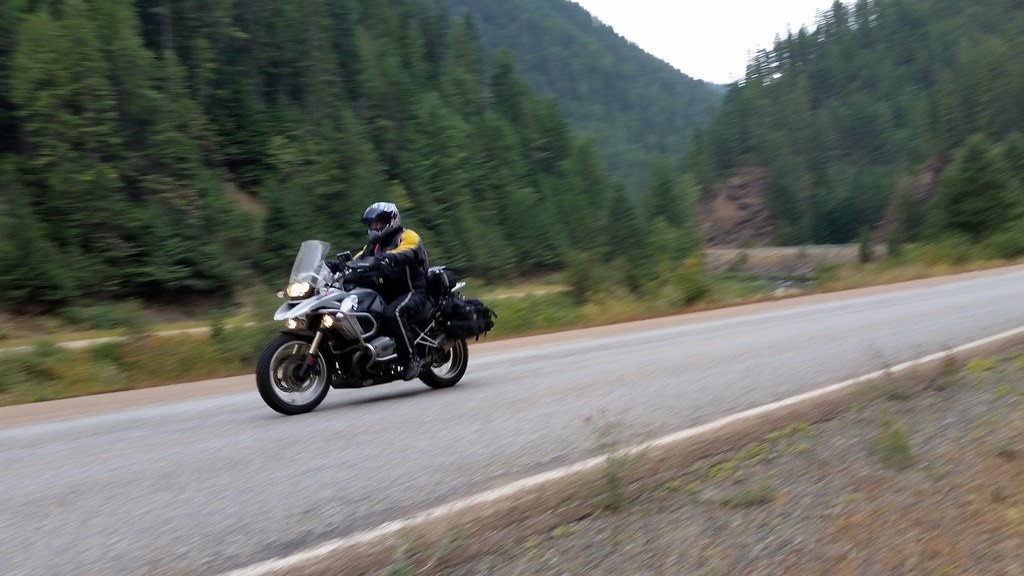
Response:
[255,239,469,416]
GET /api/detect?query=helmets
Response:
[361,200,400,244]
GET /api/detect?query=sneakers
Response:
[402,354,424,381]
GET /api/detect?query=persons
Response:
[353,202,428,381]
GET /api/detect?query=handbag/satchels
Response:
[428,266,456,295]
[441,296,497,341]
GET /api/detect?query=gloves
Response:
[347,261,377,274]
